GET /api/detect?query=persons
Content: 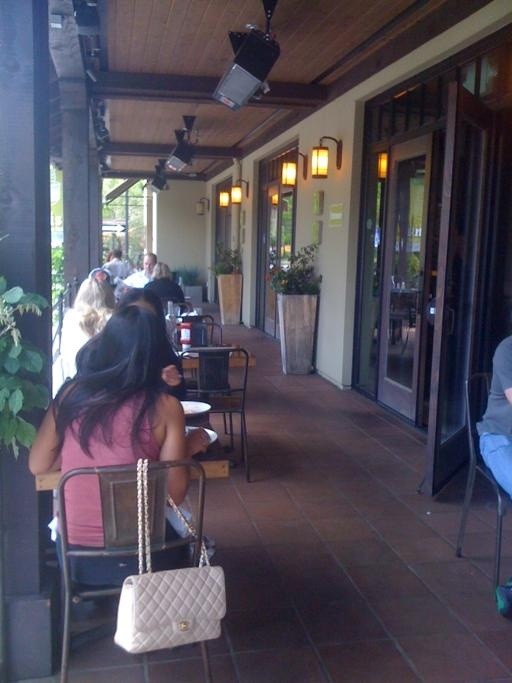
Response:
[27,247,211,589]
[476,335,512,616]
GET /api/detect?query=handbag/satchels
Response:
[111,562,229,658]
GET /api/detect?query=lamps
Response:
[165,115,199,174]
[311,136,342,179]
[219,181,230,207]
[271,188,279,206]
[377,152,389,180]
[197,198,209,215]
[231,179,248,203]
[150,159,169,194]
[280,150,307,188]
[211,0,279,112]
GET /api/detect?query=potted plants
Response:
[208,242,243,325]
[176,264,203,307]
[271,243,323,375]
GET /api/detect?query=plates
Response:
[184,424,219,449]
[179,399,212,419]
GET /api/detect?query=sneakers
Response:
[494,576,512,620]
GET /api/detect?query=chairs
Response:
[176,302,222,346]
[58,458,212,683]
[456,372,512,590]
[178,347,250,483]
